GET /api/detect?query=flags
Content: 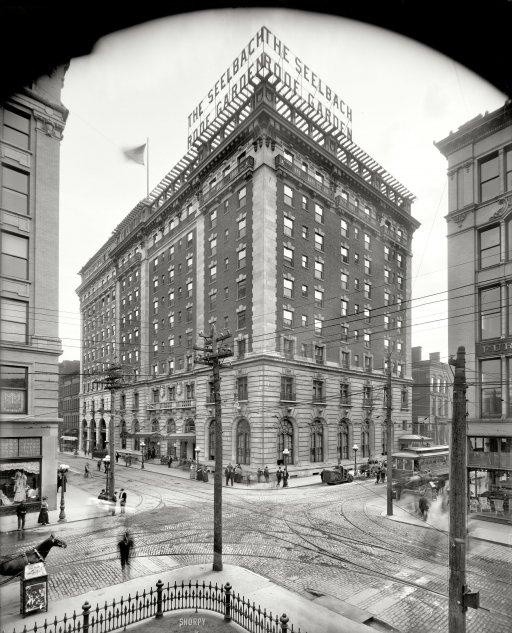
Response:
[124,144,144,166]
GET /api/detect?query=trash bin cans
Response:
[19,561,48,619]
[189,466,195,480]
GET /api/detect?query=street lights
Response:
[101,455,111,498]
[194,445,201,471]
[352,444,358,476]
[140,442,146,468]
[56,463,69,523]
[283,448,289,477]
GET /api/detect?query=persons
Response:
[374,467,383,483]
[168,455,173,468]
[128,452,134,466]
[119,489,126,513]
[381,464,387,483]
[84,463,89,473]
[146,448,151,459]
[16,500,28,530]
[114,451,119,462]
[97,459,102,471]
[38,498,48,525]
[118,533,133,571]
[225,464,288,488]
[419,494,428,512]
[151,446,155,458]
[57,474,61,493]
[124,453,128,467]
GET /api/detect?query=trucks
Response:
[320,465,353,485]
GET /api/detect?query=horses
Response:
[0,532,68,578]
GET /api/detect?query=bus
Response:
[391,445,450,490]
